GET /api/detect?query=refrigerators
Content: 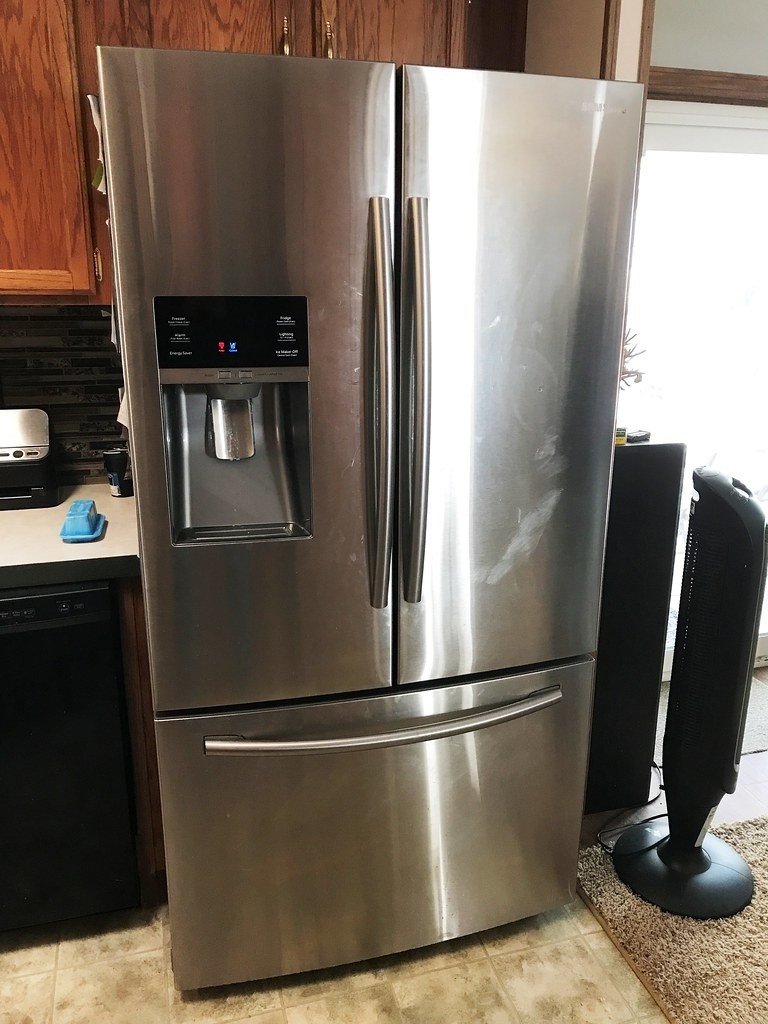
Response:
[94,44,644,994]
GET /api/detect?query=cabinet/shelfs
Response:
[0,0,529,303]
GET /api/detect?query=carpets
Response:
[576,815,768,1024]
